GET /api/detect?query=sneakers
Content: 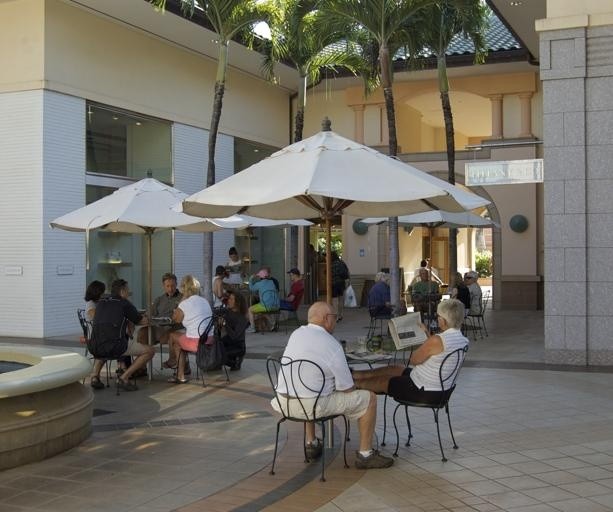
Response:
[305,438,323,463]
[246,326,257,333]
[354,448,394,469]
[267,319,276,332]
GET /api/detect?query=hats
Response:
[286,268,300,274]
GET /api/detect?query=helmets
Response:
[256,269,269,279]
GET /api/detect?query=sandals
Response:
[162,361,178,369]
[167,377,188,383]
[171,367,190,375]
[115,377,138,391]
[132,367,147,378]
[91,375,105,389]
[116,368,135,380]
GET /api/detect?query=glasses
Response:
[465,277,472,280]
[327,312,338,321]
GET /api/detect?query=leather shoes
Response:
[229,356,242,371]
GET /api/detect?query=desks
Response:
[325,352,392,451]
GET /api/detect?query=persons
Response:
[84,246,305,392]
[368,258,483,329]
[350,298,469,404]
[331,251,350,323]
[271,301,394,469]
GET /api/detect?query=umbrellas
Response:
[360,210,501,333]
[48,169,253,381]
[169,117,492,447]
[236,214,315,306]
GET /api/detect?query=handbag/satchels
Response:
[196,316,228,372]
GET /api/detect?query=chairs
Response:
[344,339,413,447]
[366,283,491,341]
[391,344,469,463]
[74,276,303,397]
[265,355,350,483]
